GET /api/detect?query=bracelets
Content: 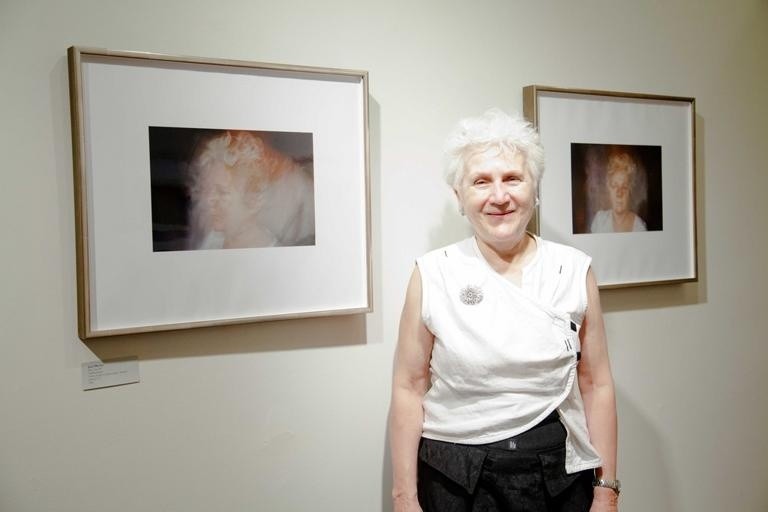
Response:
[594,478,621,494]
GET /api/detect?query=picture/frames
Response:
[65,42,377,344]
[521,83,699,292]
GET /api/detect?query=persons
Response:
[591,153,649,231]
[386,107,621,511]
[190,132,289,248]
